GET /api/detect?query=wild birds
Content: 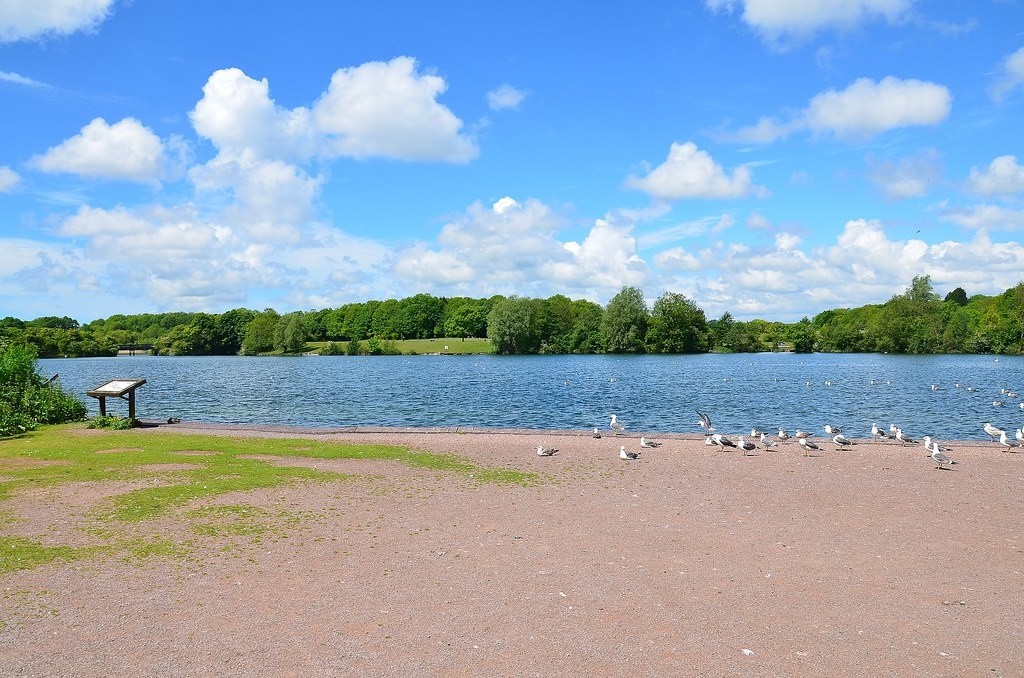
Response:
[619,445,642,460]
[609,413,625,436]
[592,428,601,439]
[983,422,1009,443]
[930,443,958,470]
[533,446,560,457]
[922,435,953,454]
[640,436,663,448]
[998,427,1024,454]
[693,408,920,458]
